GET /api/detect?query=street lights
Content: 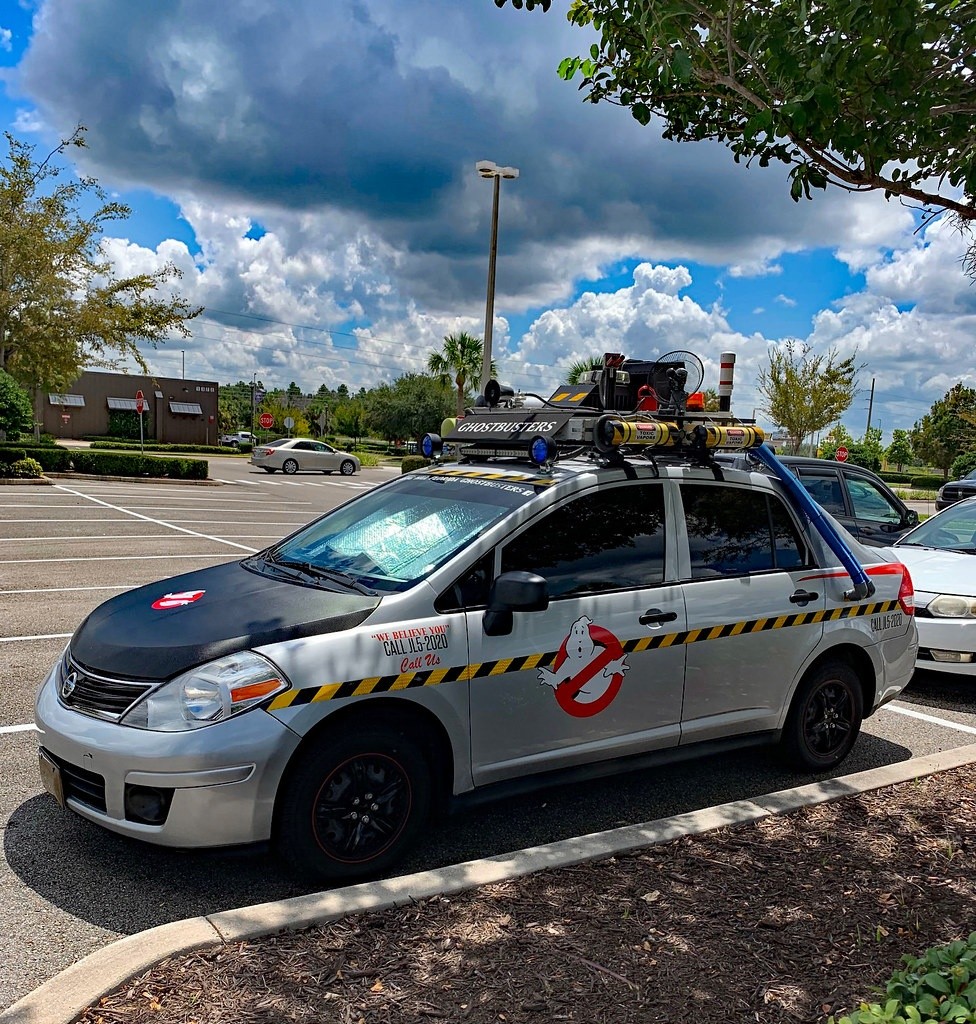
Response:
[181,350,186,381]
[475,159,520,396]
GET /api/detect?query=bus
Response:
[883,495,976,677]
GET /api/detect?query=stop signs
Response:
[259,413,275,429]
[136,390,143,415]
[836,446,849,463]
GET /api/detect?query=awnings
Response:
[169,402,202,414]
[106,397,150,410]
[48,393,85,406]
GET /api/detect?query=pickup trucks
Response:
[220,430,260,449]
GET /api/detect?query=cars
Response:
[250,437,362,476]
[33,355,924,887]
[934,469,976,515]
[710,447,960,552]
